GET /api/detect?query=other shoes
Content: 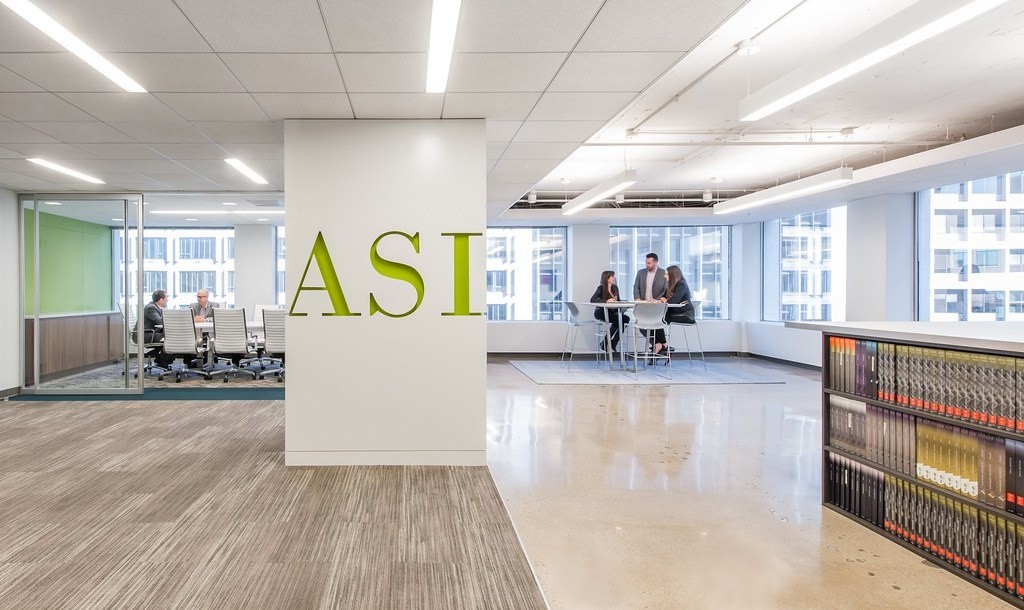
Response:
[650,344,666,363]
[649,356,657,365]
[665,356,669,365]
[665,345,675,351]
[600,343,607,352]
[154,358,174,371]
[612,346,617,353]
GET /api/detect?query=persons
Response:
[590,253,696,365]
[132,290,176,368]
[188,289,220,369]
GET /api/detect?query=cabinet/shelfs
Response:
[821,332,1024,610]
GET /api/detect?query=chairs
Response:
[158,308,209,383]
[624,303,673,380]
[561,301,608,372]
[209,307,257,383]
[663,300,708,372]
[260,309,285,382]
[240,304,282,370]
[594,310,629,370]
[117,302,172,378]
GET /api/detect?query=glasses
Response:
[196,296,206,298]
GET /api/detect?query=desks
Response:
[155,322,263,375]
[582,301,686,373]
